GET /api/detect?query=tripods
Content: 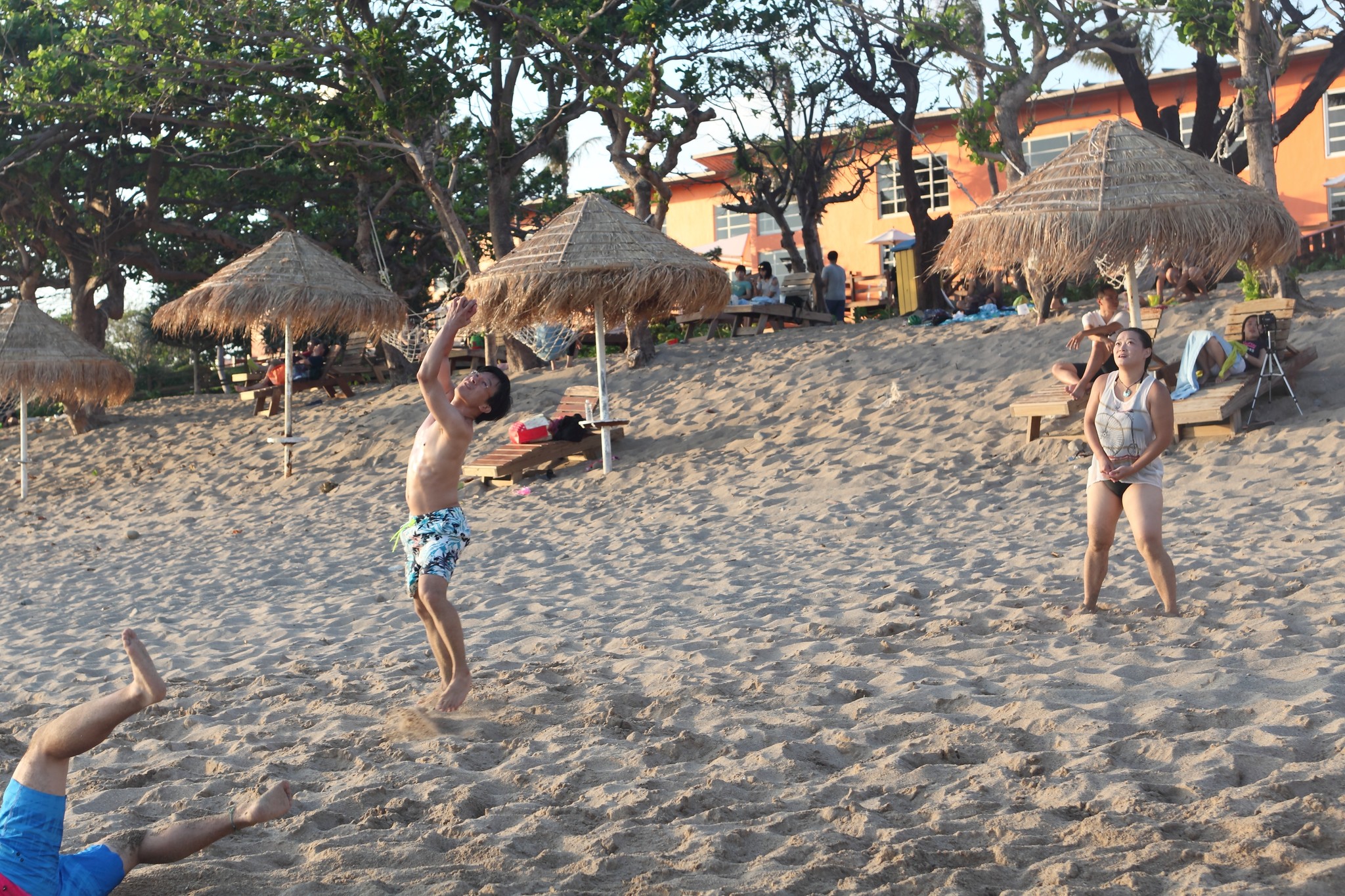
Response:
[1245,325,1304,427]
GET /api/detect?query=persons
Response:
[820,250,847,323]
[230,337,328,392]
[390,295,511,713]
[729,261,780,305]
[533,320,583,370]
[955,255,1272,400]
[1082,328,1178,618]
[0,627,293,896]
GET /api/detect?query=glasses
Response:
[758,264,765,268]
[471,369,490,397]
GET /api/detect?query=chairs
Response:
[724,272,836,337]
[674,272,761,343]
[448,327,505,375]
[1153,297,1319,443]
[232,373,266,387]
[332,331,388,384]
[240,345,356,418]
[1010,307,1166,440]
[461,384,623,485]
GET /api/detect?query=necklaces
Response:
[1118,376,1142,397]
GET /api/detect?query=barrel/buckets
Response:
[1148,294,1160,307]
[1017,303,1030,316]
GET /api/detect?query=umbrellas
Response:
[462,192,733,475]
[152,228,408,477]
[1,297,136,499]
[866,229,914,246]
[924,117,1301,328]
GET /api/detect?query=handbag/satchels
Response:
[508,413,552,444]
[267,364,285,385]
[785,295,805,325]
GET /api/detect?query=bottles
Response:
[585,399,593,421]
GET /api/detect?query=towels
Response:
[1170,329,1246,404]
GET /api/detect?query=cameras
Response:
[1258,312,1275,325]
